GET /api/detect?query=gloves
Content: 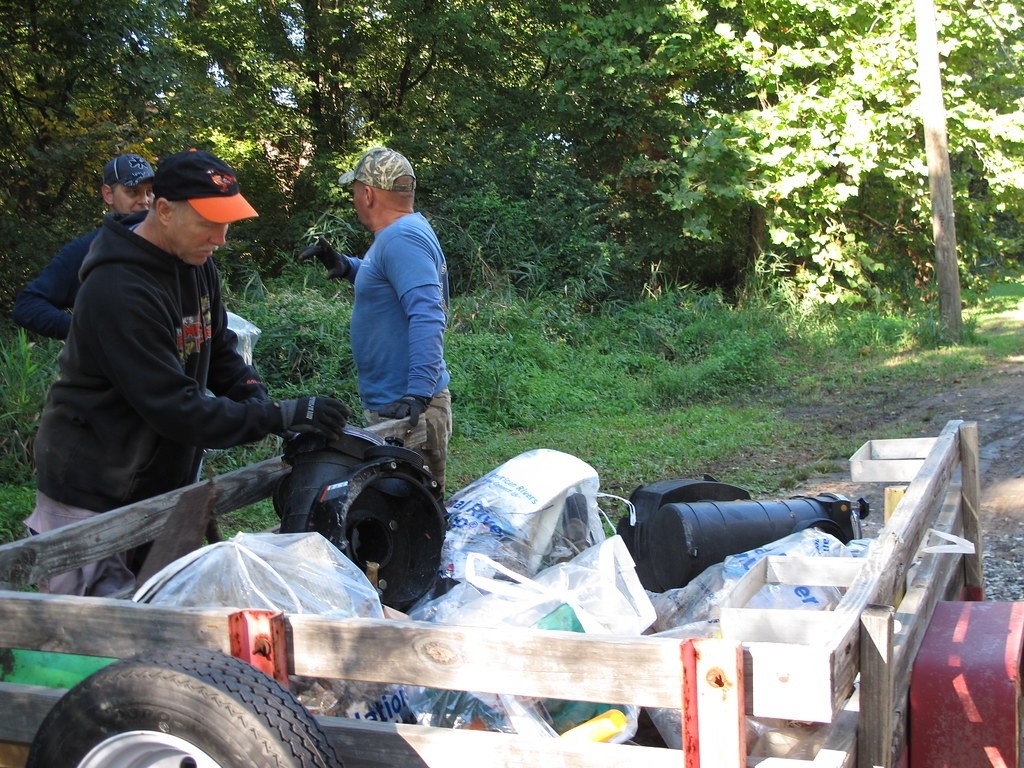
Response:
[281,396,351,440]
[379,394,432,427]
[298,235,349,280]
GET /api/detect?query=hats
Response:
[338,147,416,191]
[103,154,155,187]
[153,148,259,224]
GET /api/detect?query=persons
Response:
[12,147,351,599]
[299,147,452,504]
